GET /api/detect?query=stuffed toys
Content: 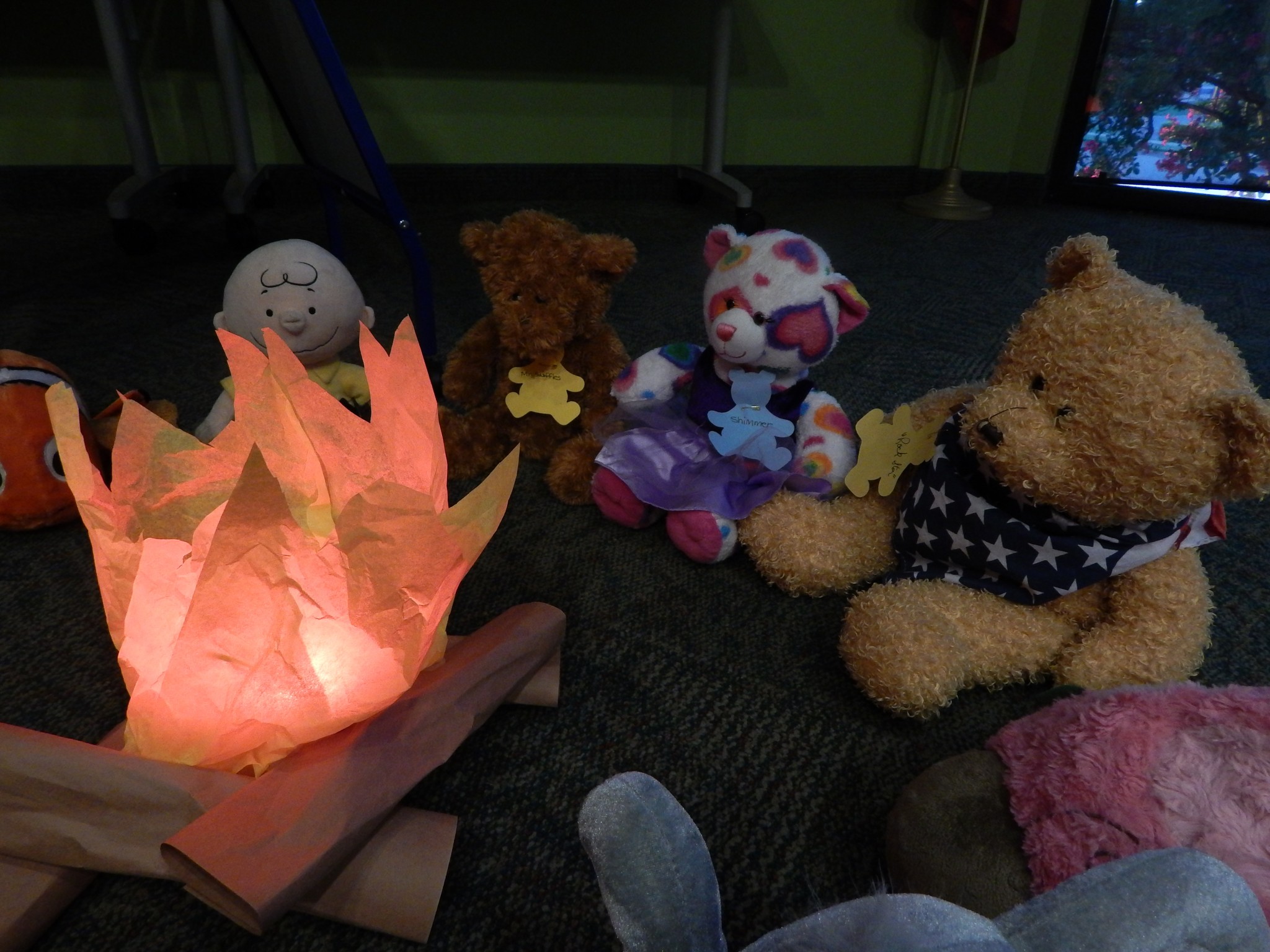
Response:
[589,221,872,563]
[736,232,1270,717]
[578,772,1270,952]
[194,238,371,446]
[0,349,177,528]
[439,210,637,503]
[985,684,1270,926]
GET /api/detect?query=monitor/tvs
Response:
[1041,0,1270,217]
